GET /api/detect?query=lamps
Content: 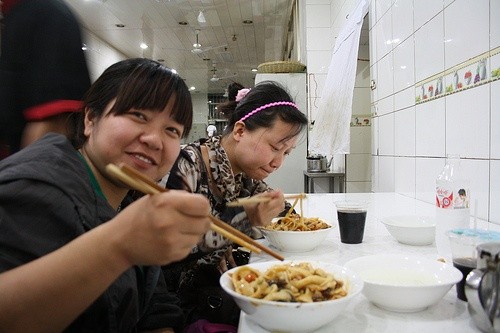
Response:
[83,44,87,50]
[223,89,228,96]
[251,68,258,71]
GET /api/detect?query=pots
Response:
[219,258,364,333]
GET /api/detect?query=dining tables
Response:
[238,193,500,333]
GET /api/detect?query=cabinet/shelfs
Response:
[254,73,308,192]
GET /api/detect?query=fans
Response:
[211,66,238,82]
[162,34,228,56]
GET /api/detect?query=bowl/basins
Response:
[256,217,332,253]
[380,212,436,246]
[345,251,463,313]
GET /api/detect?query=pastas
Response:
[231,260,347,302]
[271,195,328,231]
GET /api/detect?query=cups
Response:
[334,204,370,244]
[448,228,500,302]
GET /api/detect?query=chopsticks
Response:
[225,194,306,207]
[105,163,285,262]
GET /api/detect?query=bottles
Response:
[435,152,471,240]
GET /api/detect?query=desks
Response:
[304,172,345,193]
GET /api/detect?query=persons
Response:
[0,58,211,333]
[165,81,308,333]
[454,189,468,208]
[0,0,91,161]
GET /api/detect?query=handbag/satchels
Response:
[183,248,251,327]
